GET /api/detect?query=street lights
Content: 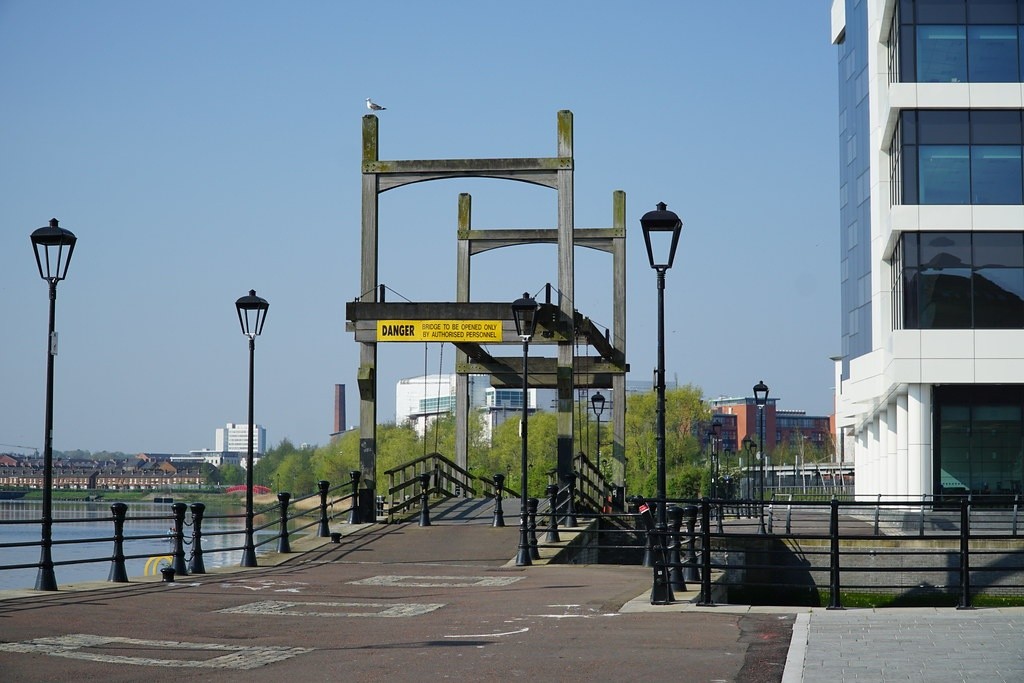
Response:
[801,435,812,494]
[591,391,605,471]
[602,459,607,477]
[277,473,280,494]
[511,292,538,566]
[639,202,684,604]
[235,289,272,567]
[712,419,721,499]
[753,380,769,534]
[750,441,757,518]
[743,436,751,519]
[30,217,79,592]
[725,448,731,475]
[507,464,511,488]
[708,431,716,498]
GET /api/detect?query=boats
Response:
[0,490,30,500]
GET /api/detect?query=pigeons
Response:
[366,98,387,114]
